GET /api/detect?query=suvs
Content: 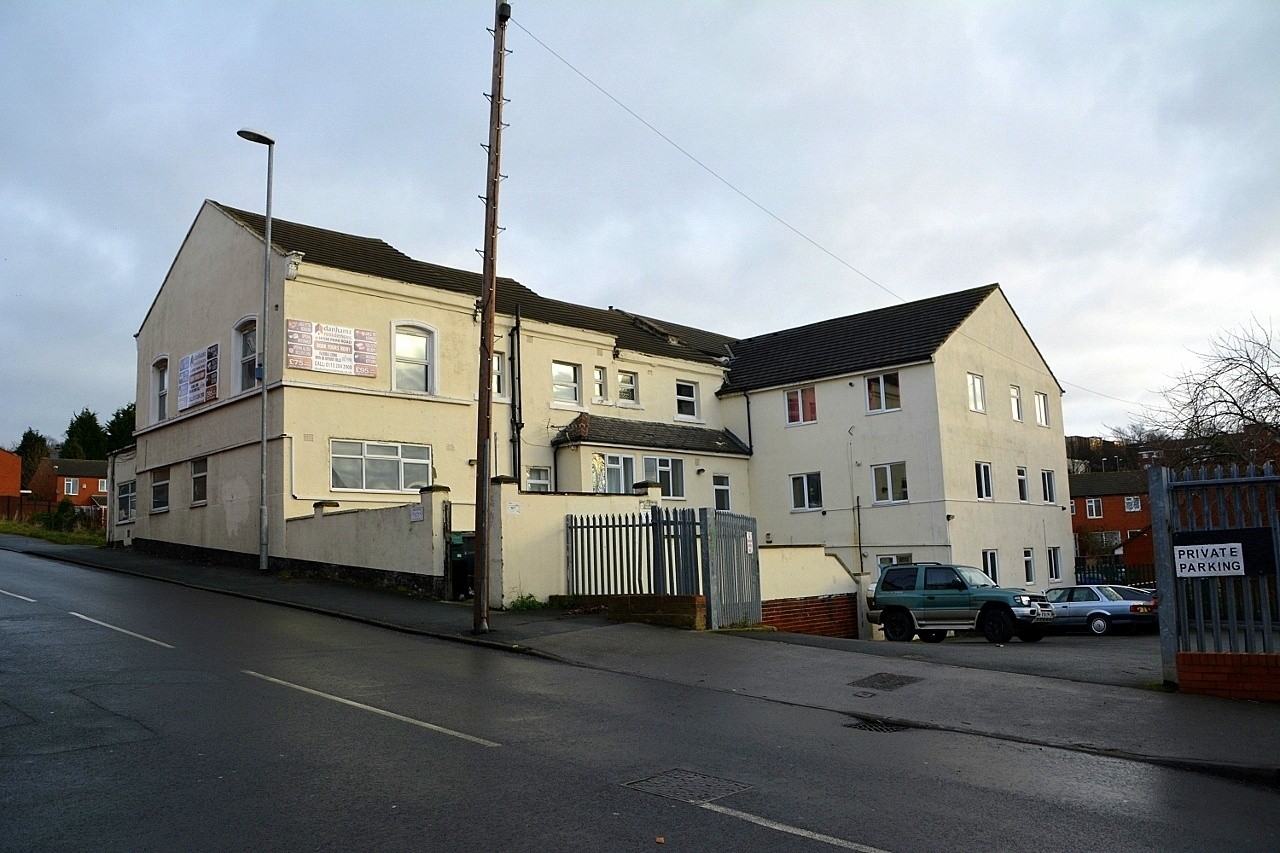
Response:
[865,561,1057,645]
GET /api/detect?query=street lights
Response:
[1101,458,1107,472]
[1113,455,1120,472]
[235,125,276,570]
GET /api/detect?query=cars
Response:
[1041,572,1160,637]
